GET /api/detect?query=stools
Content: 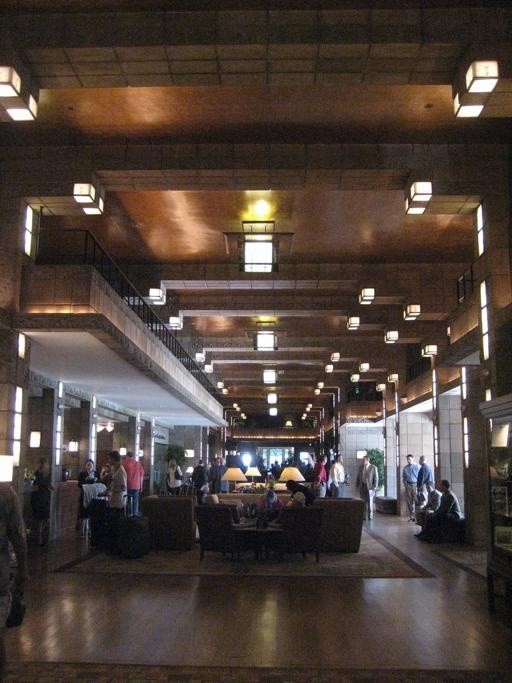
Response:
[77,487,135,549]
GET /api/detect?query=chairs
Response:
[138,483,366,560]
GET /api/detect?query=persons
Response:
[168,458,182,496]
[78,451,145,556]
[356,455,378,520]
[1,480,30,678]
[191,452,345,522]
[31,457,54,546]
[401,454,461,544]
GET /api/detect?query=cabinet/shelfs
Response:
[474,390,512,611]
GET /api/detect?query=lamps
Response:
[148,286,436,418]
[277,465,306,482]
[223,217,294,273]
[183,448,194,457]
[68,436,78,455]
[244,466,262,481]
[220,465,249,490]
[72,168,107,215]
[0,48,39,122]
[355,449,368,459]
[403,168,433,215]
[453,46,500,118]
[185,466,193,473]
[27,426,41,448]
[118,444,127,455]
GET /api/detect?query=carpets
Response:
[48,522,437,577]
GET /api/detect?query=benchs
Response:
[418,504,467,543]
[373,494,398,514]
